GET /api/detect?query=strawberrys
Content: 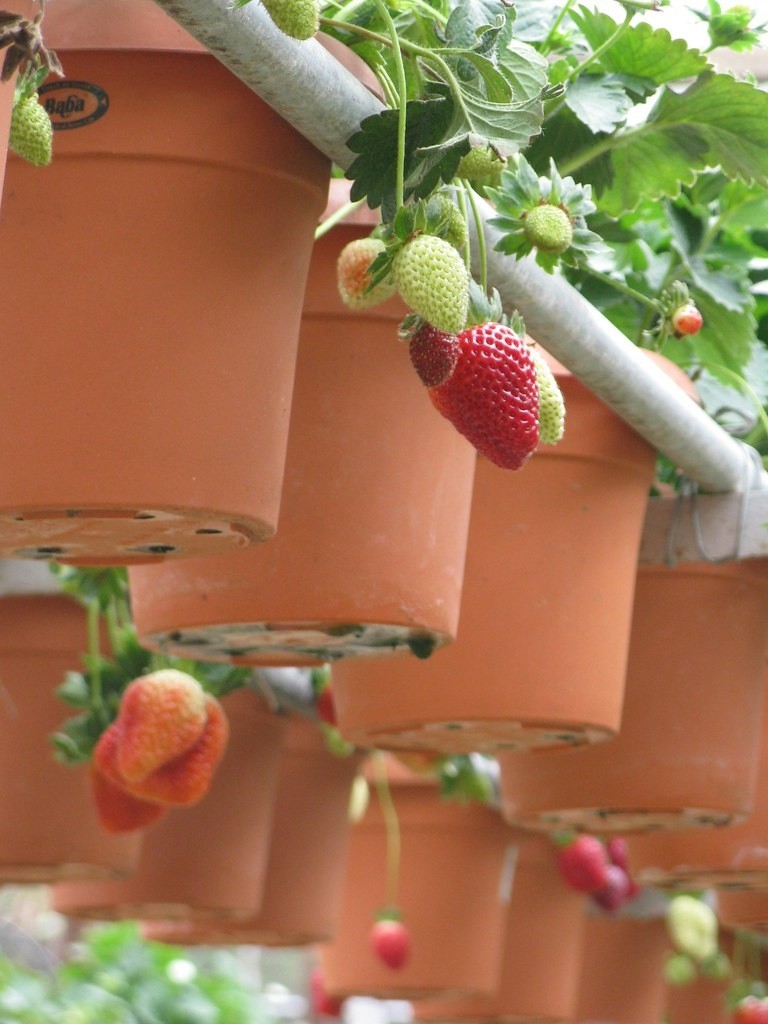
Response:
[85,309,768,1024]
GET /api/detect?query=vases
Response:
[2,0,767,1023]
